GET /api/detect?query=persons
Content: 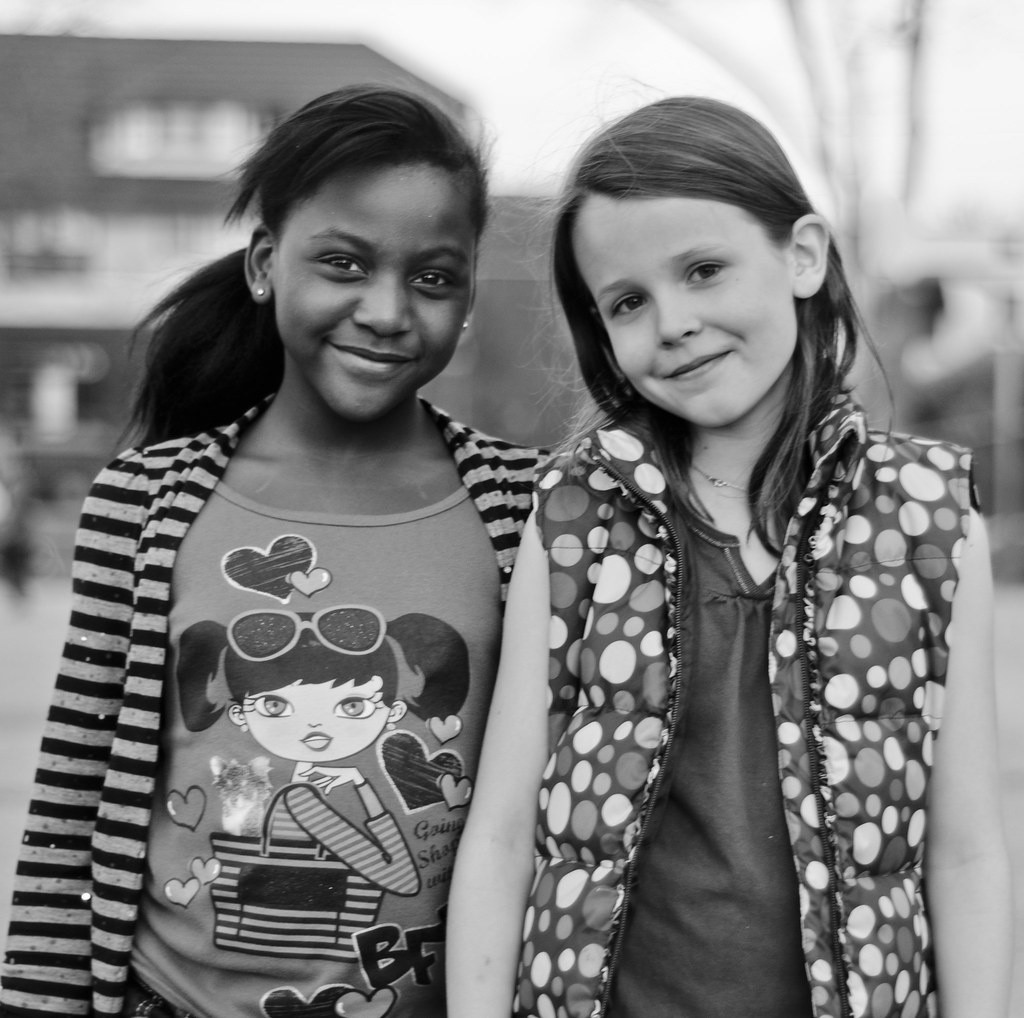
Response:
[0,89,558,1018]
[443,98,1012,1017]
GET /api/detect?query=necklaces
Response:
[692,464,751,493]
